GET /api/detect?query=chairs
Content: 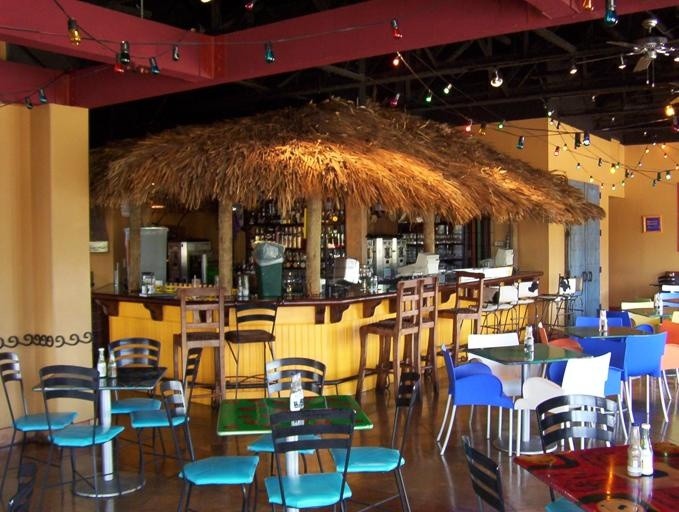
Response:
[565,325,647,427]
[378,274,442,395]
[596,308,632,328]
[37,364,125,512]
[512,352,611,451]
[247,358,327,476]
[535,394,620,512]
[546,357,623,446]
[574,315,636,427]
[503,279,540,332]
[468,333,522,448]
[541,327,583,353]
[581,332,670,425]
[459,283,518,334]
[355,277,423,410]
[541,275,583,327]
[436,343,514,457]
[223,297,283,400]
[657,322,678,344]
[101,338,170,460]
[329,372,422,512]
[426,270,485,378]
[621,302,656,329]
[522,275,576,341]
[0,352,76,496]
[161,380,260,512]
[263,408,355,512]
[129,380,197,489]
[171,286,225,420]
[633,326,672,404]
[460,434,514,512]
[658,293,678,319]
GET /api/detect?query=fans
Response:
[607,16,679,59]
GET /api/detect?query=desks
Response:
[217,394,374,512]
[32,365,169,499]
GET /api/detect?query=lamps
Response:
[38,87,50,103]
[575,132,582,148]
[676,163,679,170]
[114,54,126,73]
[66,17,82,48]
[666,171,671,181]
[583,131,591,147]
[547,106,555,117]
[645,147,649,153]
[639,161,642,167]
[612,184,616,190]
[465,118,473,133]
[22,95,35,110]
[554,144,560,155]
[390,91,401,106]
[394,54,401,66]
[497,119,505,130]
[391,17,405,41]
[172,44,181,61]
[630,172,635,179]
[568,58,578,74]
[425,91,433,102]
[653,179,657,186]
[263,41,276,63]
[517,134,525,149]
[617,51,628,71]
[616,162,620,168]
[489,66,505,90]
[622,180,625,186]
[589,175,593,183]
[664,101,675,117]
[563,143,568,151]
[598,158,602,167]
[444,82,452,94]
[550,119,554,123]
[664,153,668,159]
[556,119,562,129]
[610,162,616,174]
[657,172,662,181]
[601,183,604,189]
[119,39,132,64]
[480,122,487,135]
[577,163,580,167]
[150,55,161,75]
[652,141,657,146]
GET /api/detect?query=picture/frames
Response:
[642,215,662,232]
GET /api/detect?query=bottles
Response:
[238,276,243,302]
[295,374,305,409]
[640,423,654,476]
[599,309,607,338]
[108,350,117,378]
[524,324,534,352]
[627,423,642,477]
[289,374,300,411]
[243,276,249,300]
[252,203,345,267]
[362,279,368,295]
[98,348,106,377]
